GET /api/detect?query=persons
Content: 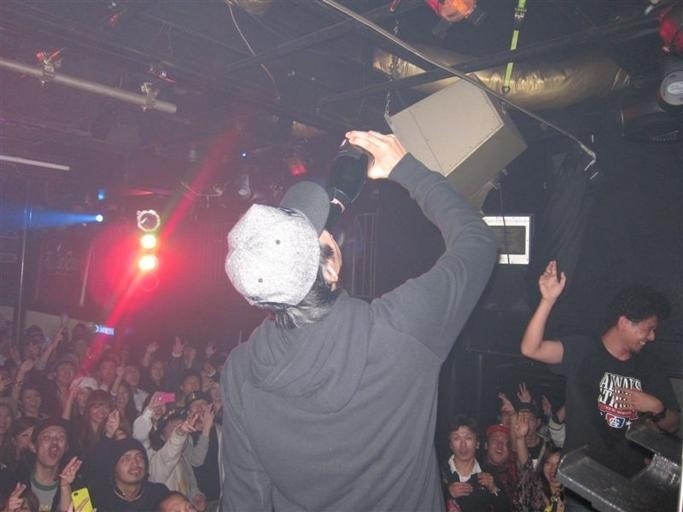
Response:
[221,129,497,512]
[440,382,570,510]
[0,314,230,511]
[520,256,681,511]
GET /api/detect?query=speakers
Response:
[385,73,528,211]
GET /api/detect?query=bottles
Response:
[325,135,374,230]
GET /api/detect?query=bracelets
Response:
[649,399,670,424]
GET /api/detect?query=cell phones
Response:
[157,391,177,404]
[70,487,93,512]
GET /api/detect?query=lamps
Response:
[95,214,107,224]
[136,205,161,274]
[655,55,683,115]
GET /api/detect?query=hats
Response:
[158,407,183,434]
[225,181,331,311]
[31,417,74,444]
[111,438,146,468]
[184,390,210,406]
